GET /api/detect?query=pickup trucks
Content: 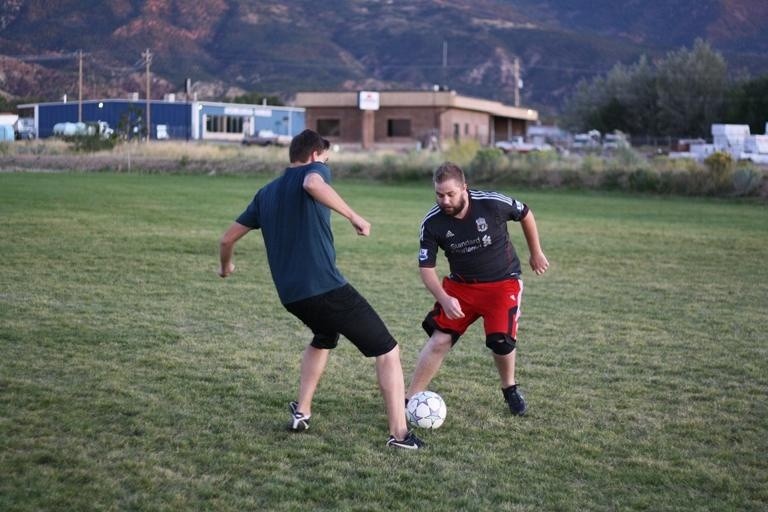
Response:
[242,128,293,147]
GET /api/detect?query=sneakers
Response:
[289,399,311,431]
[500,384,525,417]
[385,431,432,451]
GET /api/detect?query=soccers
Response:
[407,391,446,429]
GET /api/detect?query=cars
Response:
[492,126,624,156]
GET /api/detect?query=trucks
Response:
[50,119,113,140]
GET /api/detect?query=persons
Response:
[405,161,549,416]
[217,130,437,454]
